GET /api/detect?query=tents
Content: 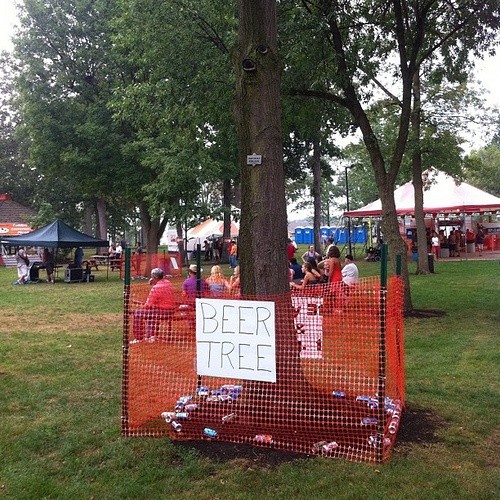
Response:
[343,166,500,260]
[0,219,110,284]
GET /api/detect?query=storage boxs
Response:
[64,267,84,282]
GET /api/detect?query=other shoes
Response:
[17,280,23,285]
[145,336,156,343]
[129,338,145,344]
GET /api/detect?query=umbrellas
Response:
[183,215,240,261]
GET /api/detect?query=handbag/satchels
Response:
[24,259,30,266]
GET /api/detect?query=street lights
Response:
[345,164,364,255]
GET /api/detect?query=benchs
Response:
[159,316,195,343]
[111,265,121,269]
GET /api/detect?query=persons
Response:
[228,267,242,299]
[15,246,29,285]
[201,237,237,268]
[74,246,84,267]
[181,264,211,330]
[129,269,176,346]
[108,241,142,276]
[288,239,358,313]
[39,246,53,283]
[374,226,486,260]
[206,264,233,299]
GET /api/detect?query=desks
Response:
[90,255,108,266]
[110,259,121,272]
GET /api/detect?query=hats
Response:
[185,263,204,272]
[344,254,354,260]
